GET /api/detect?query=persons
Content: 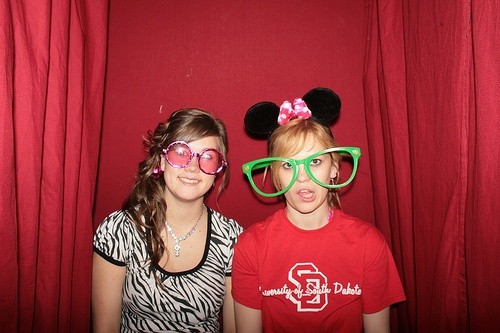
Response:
[232,87,406,333]
[93,108,245,333]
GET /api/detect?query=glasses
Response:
[163,141,228,175]
[242,147,362,197]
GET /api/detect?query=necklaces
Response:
[164,201,204,256]
